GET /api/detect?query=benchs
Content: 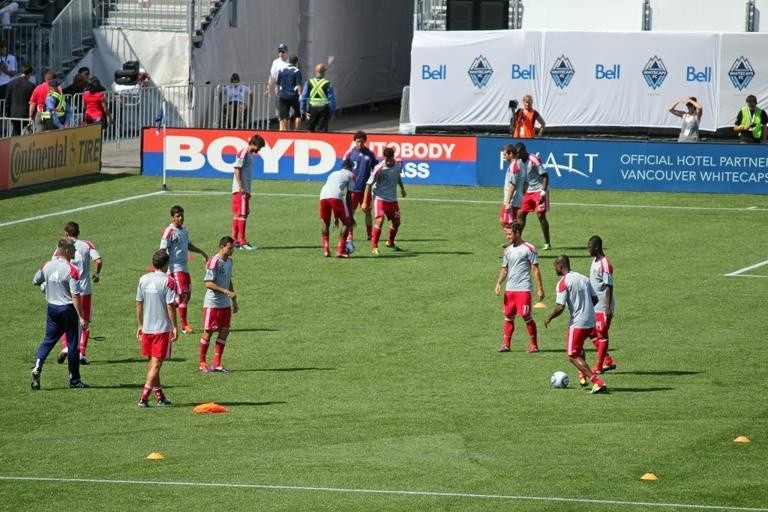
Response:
[11,0,224,91]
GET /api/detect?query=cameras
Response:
[748,122,756,128]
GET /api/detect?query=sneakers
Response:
[233,242,239,249]
[499,346,511,352]
[210,367,230,373]
[323,232,400,257]
[239,244,257,250]
[138,399,153,407]
[157,399,172,406]
[579,361,616,394]
[30,351,90,389]
[180,324,193,333]
[542,244,551,251]
[526,346,538,353]
[199,365,209,372]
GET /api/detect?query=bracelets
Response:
[740,126,744,130]
[605,303,610,308]
[538,132,542,136]
[224,288,229,295]
[95,271,99,278]
[201,251,206,257]
[540,190,547,196]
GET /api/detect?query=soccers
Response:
[40,281,47,294]
[551,371,569,388]
[345,240,355,254]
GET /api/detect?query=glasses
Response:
[279,50,285,53]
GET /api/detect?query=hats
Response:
[277,44,288,51]
[48,80,60,87]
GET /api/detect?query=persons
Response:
[669,97,703,144]
[509,95,545,139]
[588,235,616,374]
[0,45,114,136]
[31,238,89,389]
[219,73,253,130]
[734,95,768,144]
[137,250,178,407]
[544,255,607,393]
[159,205,209,334]
[264,43,407,258]
[230,134,265,251]
[499,144,529,248]
[515,142,552,250]
[51,221,102,365]
[495,222,545,353]
[198,236,238,372]
